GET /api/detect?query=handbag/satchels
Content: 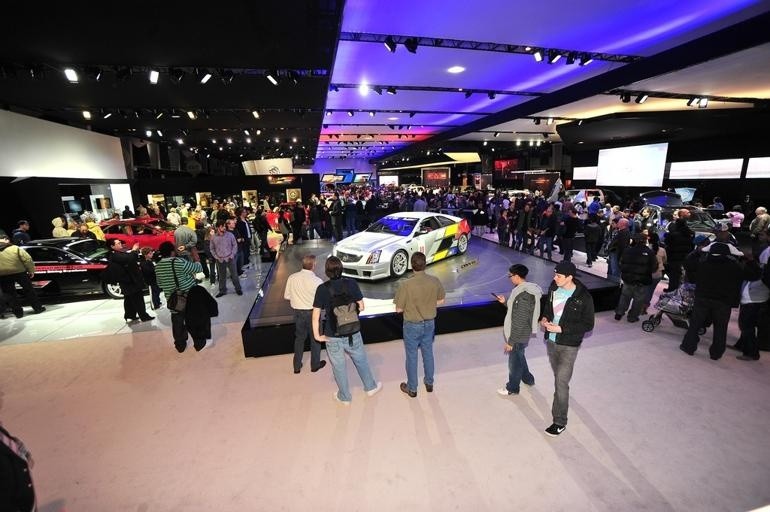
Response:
[166,289,190,314]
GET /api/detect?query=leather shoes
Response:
[310,359,327,373]
[293,361,303,375]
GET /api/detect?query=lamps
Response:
[383,36,397,53]
[699,98,708,108]
[581,58,594,66]
[525,46,533,52]
[533,51,543,62]
[687,97,700,106]
[549,52,561,63]
[565,52,576,64]
[635,94,648,104]
[36,64,595,161]
[404,40,419,54]
[622,95,630,103]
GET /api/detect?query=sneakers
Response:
[236,290,244,295]
[710,356,719,361]
[679,344,695,355]
[399,380,418,398]
[496,385,521,396]
[544,422,568,438]
[238,273,248,280]
[332,390,353,407]
[725,343,738,350]
[35,305,46,314]
[735,354,760,361]
[626,317,641,323]
[423,375,434,393]
[214,292,226,298]
[364,380,384,398]
[613,314,623,321]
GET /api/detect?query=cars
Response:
[327,210,474,280]
[0,427,36,511]
[10,218,187,299]
[502,189,534,199]
[264,202,310,229]
[400,183,427,194]
[321,193,366,210]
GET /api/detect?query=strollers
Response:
[641,263,709,337]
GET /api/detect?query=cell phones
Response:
[491,292,499,299]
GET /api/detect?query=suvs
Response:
[563,188,607,214]
[639,188,739,251]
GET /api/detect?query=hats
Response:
[508,263,529,279]
[691,234,711,245]
[553,259,582,278]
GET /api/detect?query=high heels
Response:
[141,316,156,323]
[123,314,140,323]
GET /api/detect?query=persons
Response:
[495,263,543,397]
[539,259,595,437]
[0,392,41,512]
[392,249,445,397]
[311,254,385,405]
[282,254,328,373]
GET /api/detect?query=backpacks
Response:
[321,276,363,338]
